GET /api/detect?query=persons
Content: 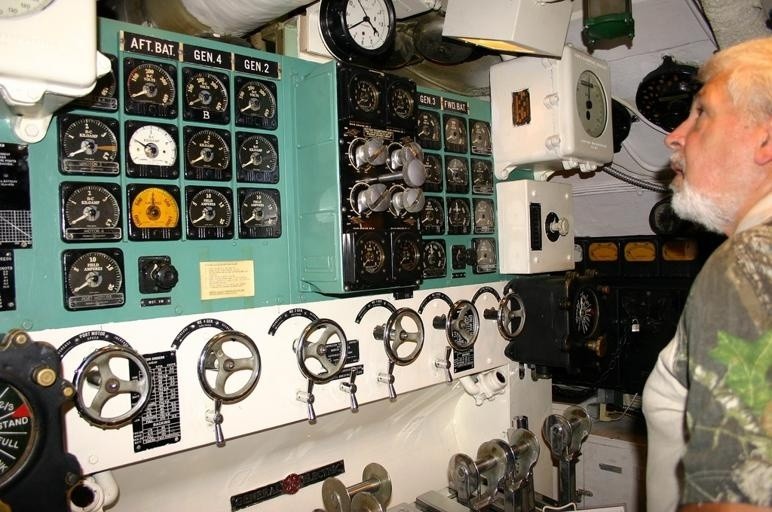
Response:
[638,35,772,512]
